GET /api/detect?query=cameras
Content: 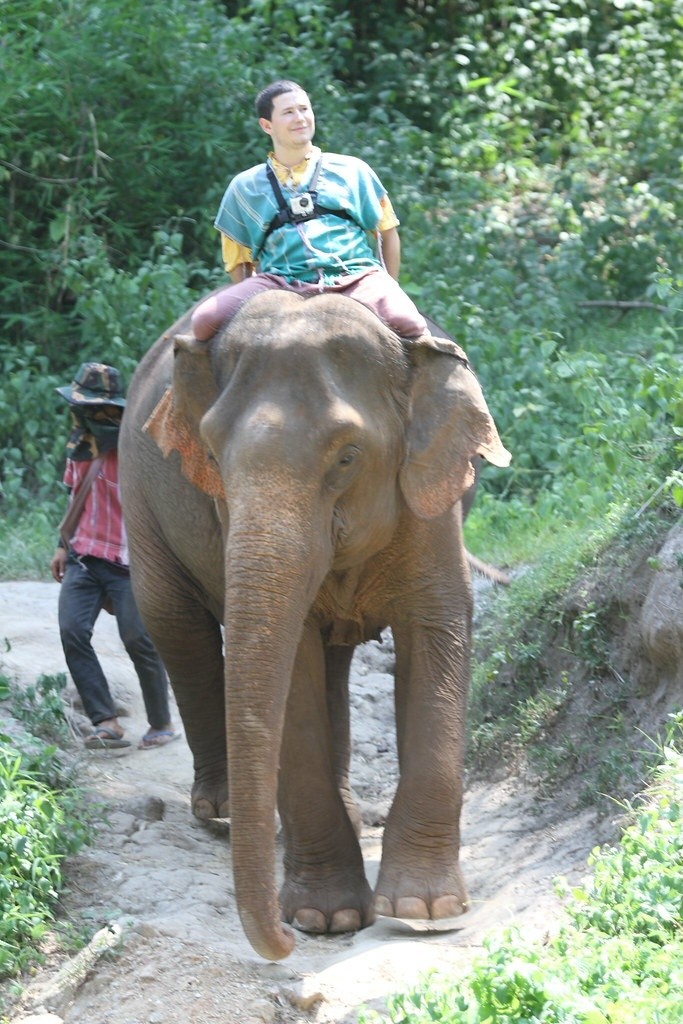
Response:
[290,192,314,216]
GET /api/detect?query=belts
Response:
[71,552,130,576]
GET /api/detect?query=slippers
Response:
[85,727,131,749]
[136,730,181,749]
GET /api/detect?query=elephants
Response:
[119,283,513,963]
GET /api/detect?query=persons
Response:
[46,363,181,752]
[192,80,430,341]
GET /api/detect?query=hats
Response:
[53,363,126,407]
